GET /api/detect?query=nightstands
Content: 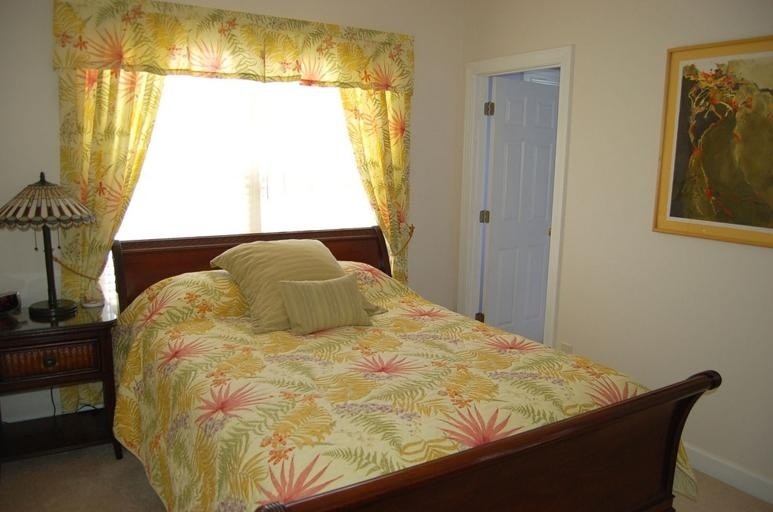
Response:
[119,233,722,512]
[1,303,122,460]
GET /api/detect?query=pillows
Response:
[275,271,372,337]
[140,261,421,331]
[210,238,388,335]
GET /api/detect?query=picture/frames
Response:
[652,34,773,248]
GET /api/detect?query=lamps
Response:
[0,171,96,323]
